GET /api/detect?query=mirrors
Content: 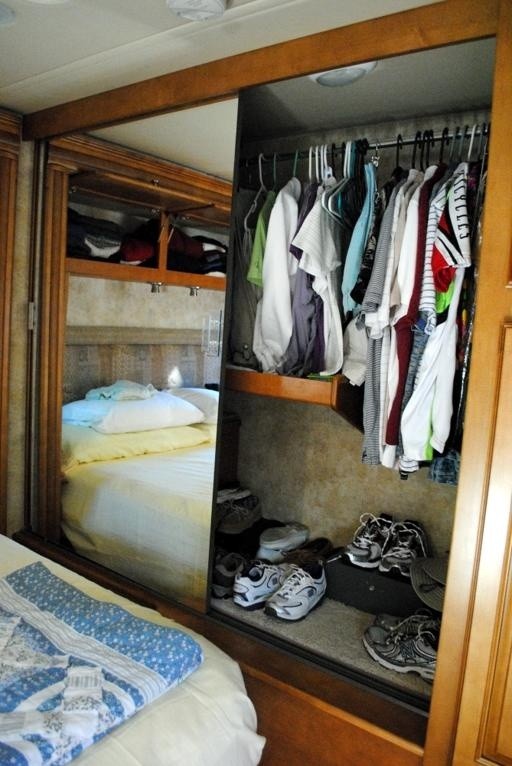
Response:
[24,92,244,618]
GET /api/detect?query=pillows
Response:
[167,388,219,425]
[62,423,212,474]
[189,423,218,442]
[62,389,207,434]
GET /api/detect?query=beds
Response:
[1,533,268,766]
[61,379,220,617]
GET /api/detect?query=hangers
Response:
[244,121,493,236]
